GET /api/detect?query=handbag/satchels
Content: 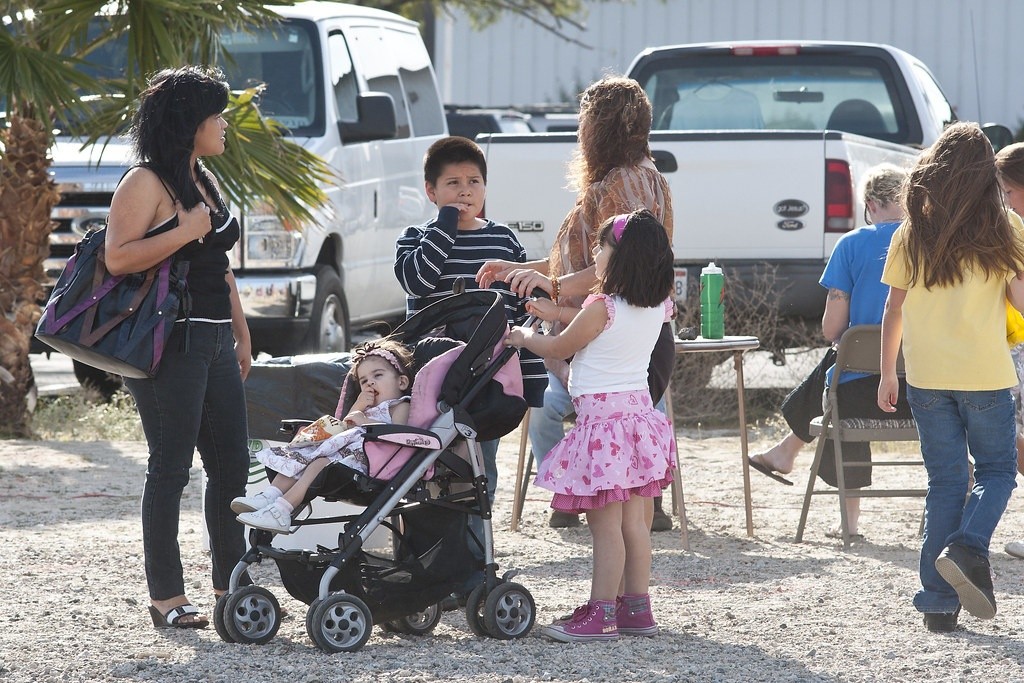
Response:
[34,158,204,379]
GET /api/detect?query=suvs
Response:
[443,102,586,143]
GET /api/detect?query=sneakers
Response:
[235,504,292,535]
[544,600,621,642]
[230,492,272,514]
[615,593,659,636]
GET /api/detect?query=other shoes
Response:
[652,507,672,531]
[935,542,997,619]
[924,602,962,632]
[550,509,580,527]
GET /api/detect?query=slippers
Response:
[826,524,865,539]
[744,455,794,486]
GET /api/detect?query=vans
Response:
[0,0,454,404]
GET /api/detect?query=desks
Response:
[505,337,759,551]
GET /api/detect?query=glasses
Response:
[864,204,873,225]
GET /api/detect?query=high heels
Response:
[149,604,210,629]
[214,592,288,620]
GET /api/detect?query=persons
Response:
[230,331,418,535]
[995,142,1024,558]
[105,62,251,628]
[503,200,675,641]
[395,136,550,612]
[877,118,1024,634]
[528,355,673,532]
[747,162,908,540]
[476,61,674,408]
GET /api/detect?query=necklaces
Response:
[882,217,905,223]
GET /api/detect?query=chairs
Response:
[793,326,931,550]
[825,99,889,139]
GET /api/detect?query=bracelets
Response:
[557,306,565,321]
[550,275,561,306]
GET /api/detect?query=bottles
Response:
[699,262,725,339]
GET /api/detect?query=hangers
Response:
[693,75,734,94]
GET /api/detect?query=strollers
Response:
[211,278,553,655]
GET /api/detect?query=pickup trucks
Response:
[472,41,959,322]
